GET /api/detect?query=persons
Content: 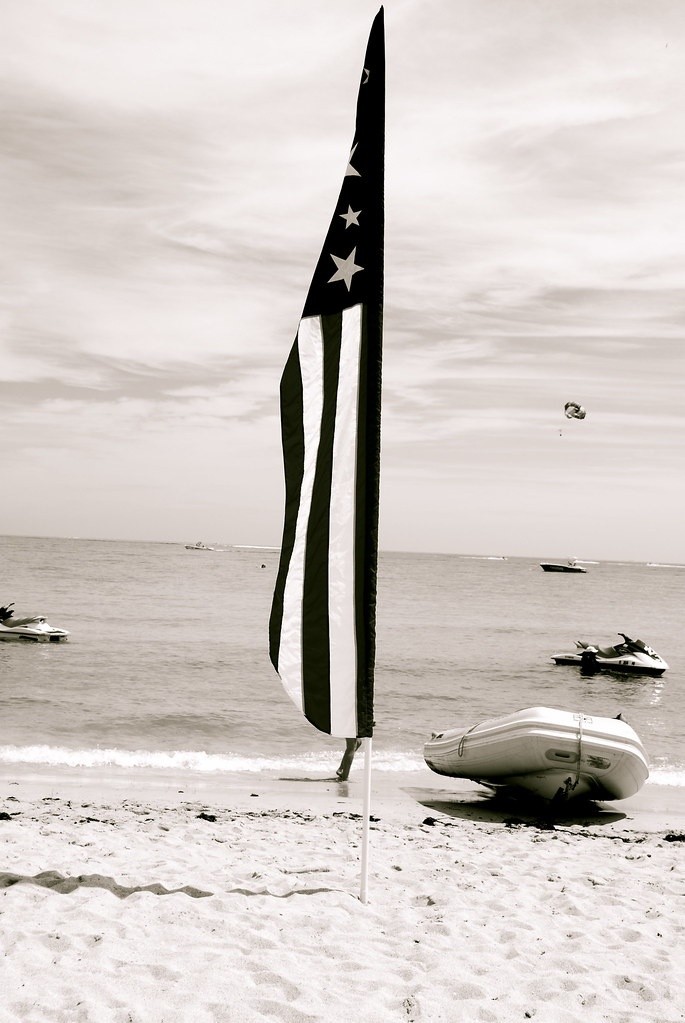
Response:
[336,738,362,780]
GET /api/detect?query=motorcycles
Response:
[0,602,72,644]
[548,632,671,677]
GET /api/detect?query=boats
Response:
[539,560,587,574]
[184,540,215,551]
[424,706,651,810]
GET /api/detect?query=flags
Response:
[265,12,386,739]
